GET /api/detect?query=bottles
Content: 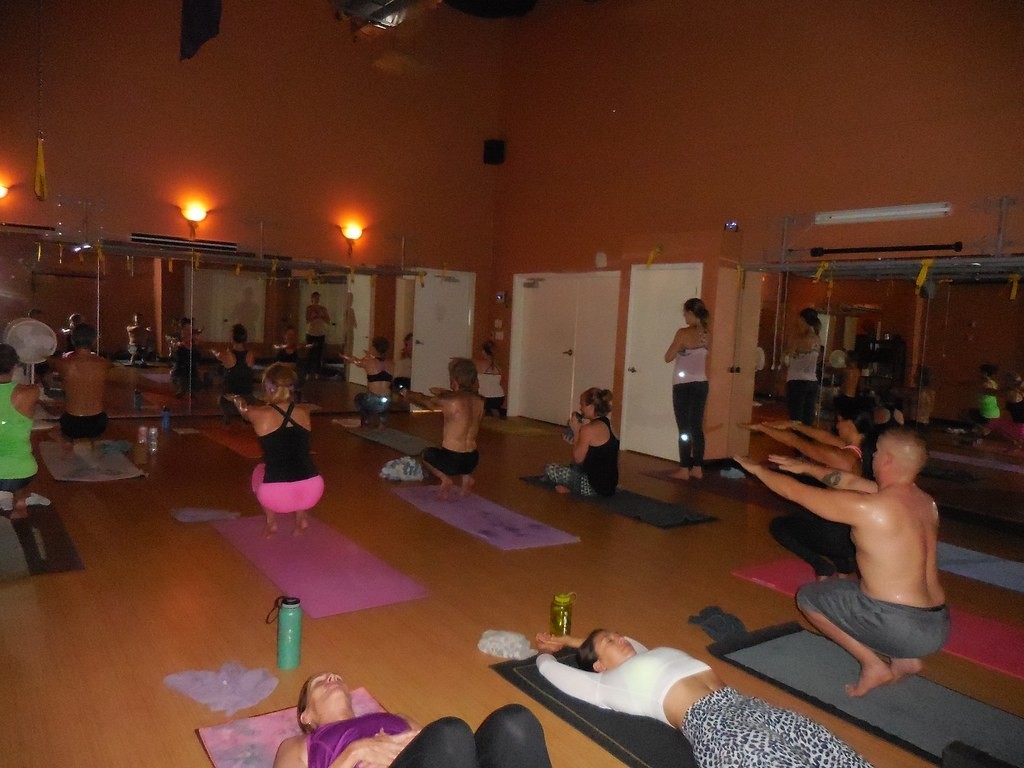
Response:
[331,417,362,428]
[565,411,585,440]
[551,591,577,636]
[135,426,158,466]
[134,389,142,412]
[266,597,302,671]
[163,406,171,429]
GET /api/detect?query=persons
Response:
[829,349,860,415]
[884,365,935,430]
[0,344,40,519]
[210,324,266,424]
[451,341,506,421]
[665,298,711,480]
[730,427,951,697]
[543,387,620,498]
[398,358,483,495]
[125,312,209,407]
[535,629,876,768]
[779,306,823,458]
[392,333,413,391]
[224,363,325,539]
[46,323,177,448]
[338,336,393,430]
[28,308,81,388]
[845,386,905,473]
[738,407,879,585]
[273,671,552,768]
[275,291,331,394]
[962,363,1024,438]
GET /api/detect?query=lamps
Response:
[813,201,952,226]
[183,205,207,236]
[340,223,362,254]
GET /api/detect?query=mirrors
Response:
[747,270,1024,526]
[1,230,417,420]
